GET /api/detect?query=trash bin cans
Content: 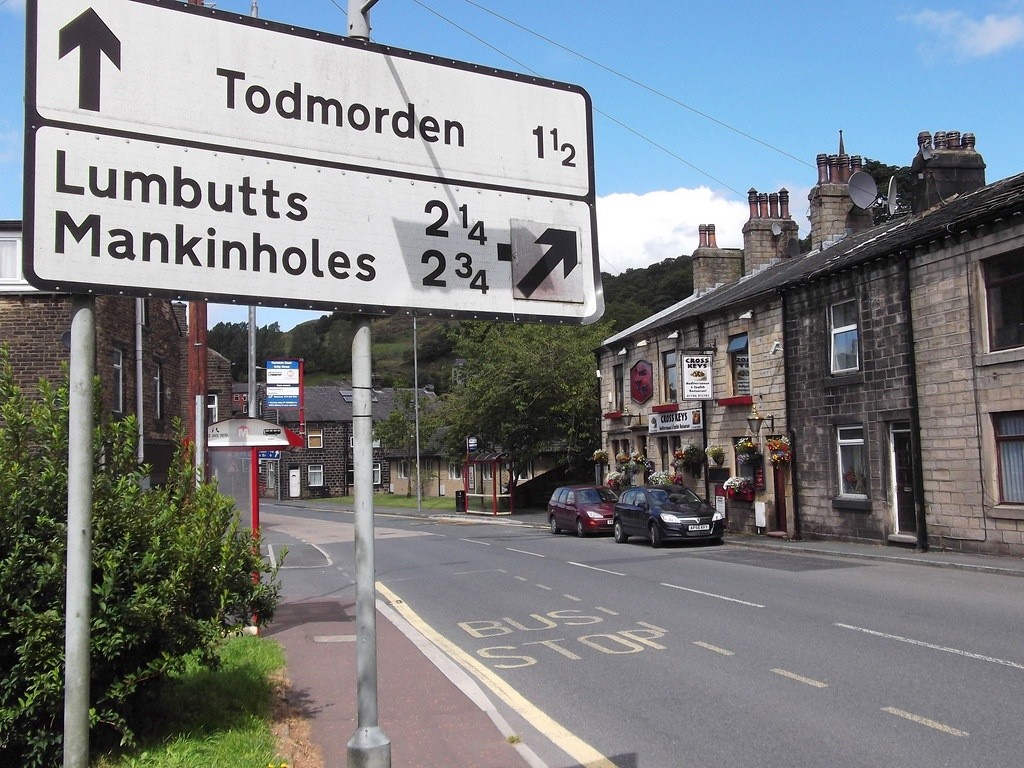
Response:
[456,490,466,512]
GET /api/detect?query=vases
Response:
[726,485,755,502]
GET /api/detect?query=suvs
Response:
[546,485,621,538]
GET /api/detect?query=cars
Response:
[614,483,726,548]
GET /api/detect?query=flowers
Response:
[734,437,760,465]
[646,472,683,486]
[764,434,793,470]
[604,472,629,496]
[615,451,653,473]
[670,443,705,470]
[704,444,725,465]
[722,478,753,494]
[593,449,608,466]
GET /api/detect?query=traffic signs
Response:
[23,0,604,327]
